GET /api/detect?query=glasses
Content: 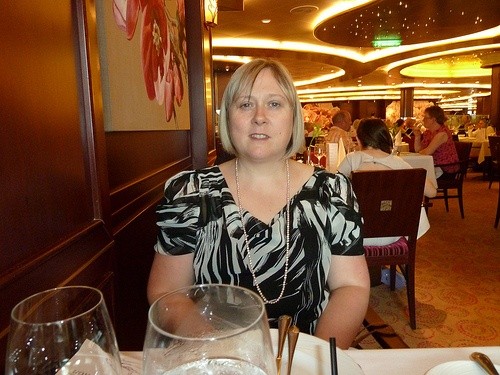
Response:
[421,115,431,121]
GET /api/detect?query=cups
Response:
[142,283,277,375]
[4,285,124,375]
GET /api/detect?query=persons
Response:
[349,109,497,147]
[412,106,460,181]
[337,118,414,288]
[327,110,358,153]
[148,57,371,350]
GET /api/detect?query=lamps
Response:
[203,0,218,31]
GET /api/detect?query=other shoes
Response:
[380,268,406,289]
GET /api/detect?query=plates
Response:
[150,325,366,375]
[423,358,500,375]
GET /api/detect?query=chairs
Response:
[424,142,472,219]
[350,168,427,330]
[483,136,500,189]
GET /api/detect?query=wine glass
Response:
[314,144,325,167]
[404,120,423,139]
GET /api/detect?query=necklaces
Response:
[235,158,290,304]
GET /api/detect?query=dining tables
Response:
[392,152,438,198]
[458,136,491,178]
